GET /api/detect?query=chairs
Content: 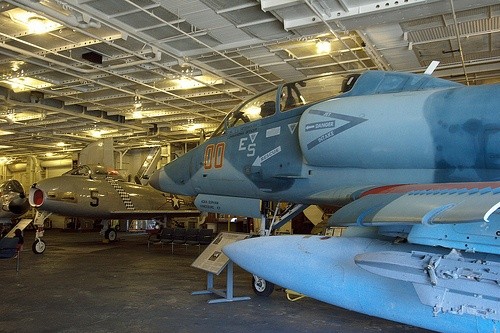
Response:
[148,227,213,254]
[0,237,20,287]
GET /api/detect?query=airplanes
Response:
[147,70,500,333]
[28,164,202,254]
